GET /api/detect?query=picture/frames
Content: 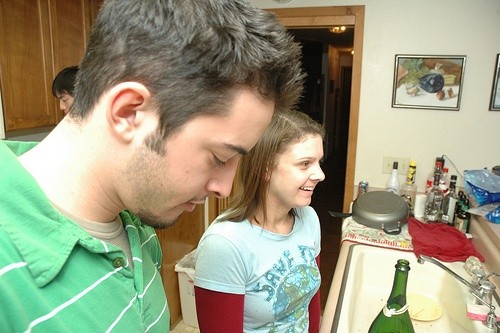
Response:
[392,54,467,111]
[488,53,500,111]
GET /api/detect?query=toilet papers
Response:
[413,194,431,221]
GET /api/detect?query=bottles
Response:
[398,160,417,219]
[452,191,470,235]
[439,168,449,196]
[440,175,458,226]
[367,258,415,333]
[424,168,444,224]
[425,162,442,195]
[386,161,401,196]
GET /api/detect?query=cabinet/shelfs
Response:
[0,0,205,330]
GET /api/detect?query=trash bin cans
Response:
[174,249,199,329]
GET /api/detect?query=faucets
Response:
[417,253,496,305]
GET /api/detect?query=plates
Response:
[405,292,443,322]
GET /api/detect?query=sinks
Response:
[330,244,477,333]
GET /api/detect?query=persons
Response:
[0,0,300,333]
[53,66,79,114]
[193,111,326,333]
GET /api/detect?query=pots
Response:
[329,191,411,235]
